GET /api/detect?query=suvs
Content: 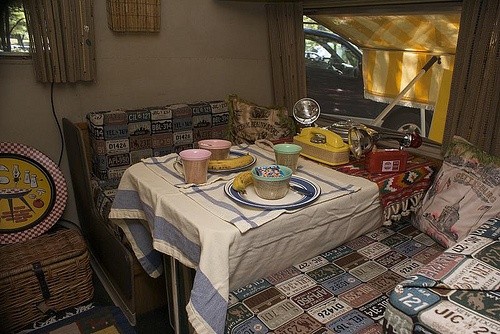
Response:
[303,28,435,139]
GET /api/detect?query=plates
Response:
[207,151,257,172]
[224,175,321,209]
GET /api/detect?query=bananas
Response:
[208,153,254,170]
[232,171,253,192]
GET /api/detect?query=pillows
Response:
[225,94,296,144]
[410,136,500,249]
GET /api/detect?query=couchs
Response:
[224,211,500,334]
[62,95,318,326]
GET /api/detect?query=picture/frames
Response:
[0,142,67,244]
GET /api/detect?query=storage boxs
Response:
[106,0,161,33]
[364,146,409,174]
[0,227,95,334]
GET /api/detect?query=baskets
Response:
[0,225,95,334]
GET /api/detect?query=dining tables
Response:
[108,143,443,334]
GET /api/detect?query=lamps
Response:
[292,98,321,124]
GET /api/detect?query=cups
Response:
[273,143,302,173]
[173,149,211,184]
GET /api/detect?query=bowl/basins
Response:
[251,165,293,200]
[198,139,231,161]
